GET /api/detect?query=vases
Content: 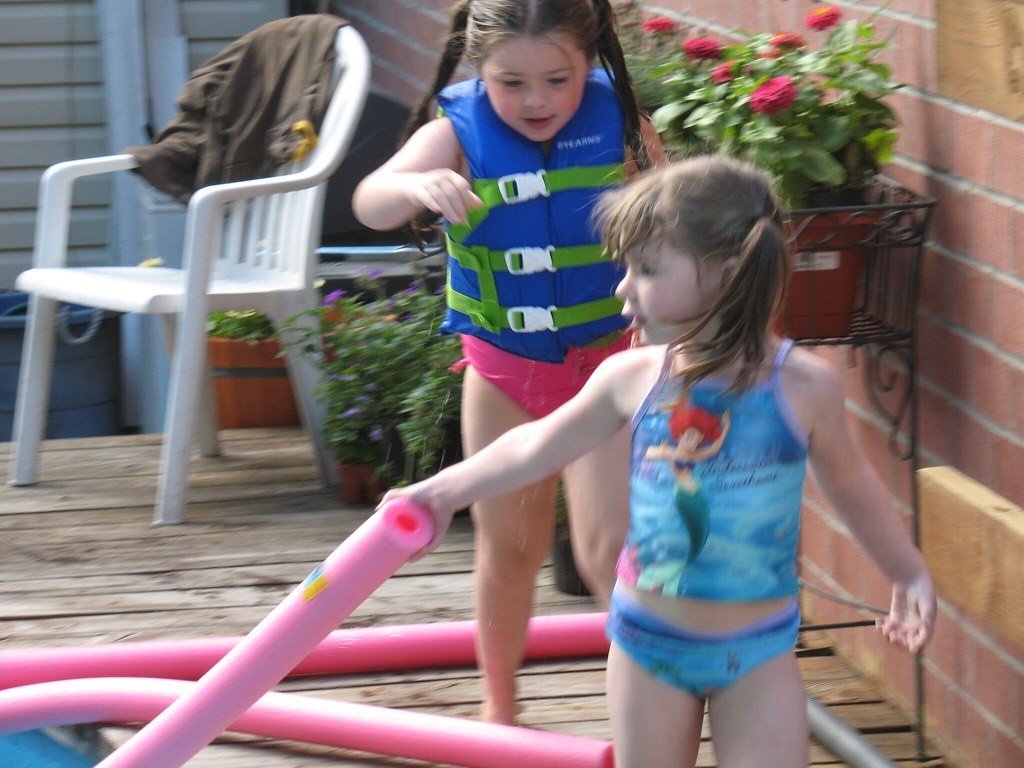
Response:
[334,463,371,503]
[764,212,878,338]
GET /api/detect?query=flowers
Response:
[269,268,461,460]
[200,279,340,339]
[639,0,910,223]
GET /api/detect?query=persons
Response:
[350,0,665,721]
[375,159,937,767]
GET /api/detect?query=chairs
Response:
[7,14,371,525]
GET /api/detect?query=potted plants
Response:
[208,335,334,429]
